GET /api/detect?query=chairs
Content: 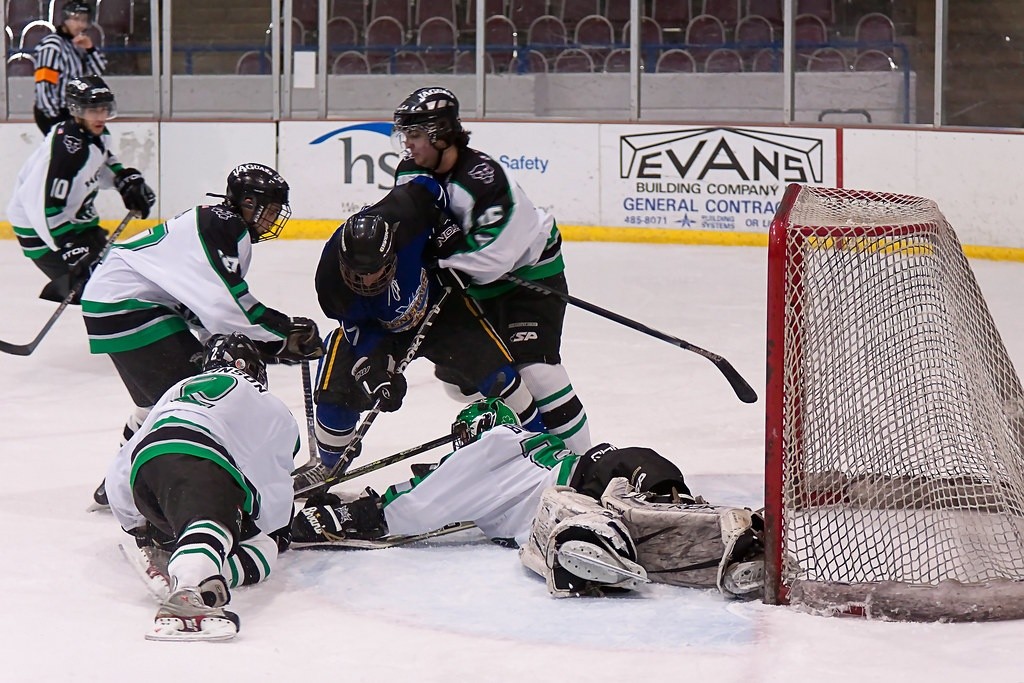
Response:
[0,0,918,76]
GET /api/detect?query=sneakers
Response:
[557,540,653,590]
[116,542,173,602]
[145,588,240,640]
[83,478,111,512]
[722,552,804,593]
[291,463,344,500]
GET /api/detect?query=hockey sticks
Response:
[294,372,507,500]
[291,360,317,476]
[291,521,478,550]
[498,273,758,404]
[0,210,137,356]
[319,286,452,493]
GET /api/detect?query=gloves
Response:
[435,265,472,303]
[284,317,324,360]
[112,167,157,219]
[350,355,407,412]
[254,348,302,365]
[289,499,360,541]
[53,242,99,273]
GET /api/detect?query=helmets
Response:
[64,76,116,120]
[451,397,523,452]
[61,0,93,24]
[394,87,459,141]
[227,164,290,243]
[202,332,267,386]
[338,214,398,295]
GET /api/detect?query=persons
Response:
[286,394,803,600]
[106,332,301,642]
[6,76,157,283]
[292,176,545,503]
[32,0,107,136]
[395,86,593,451]
[89,163,327,513]
